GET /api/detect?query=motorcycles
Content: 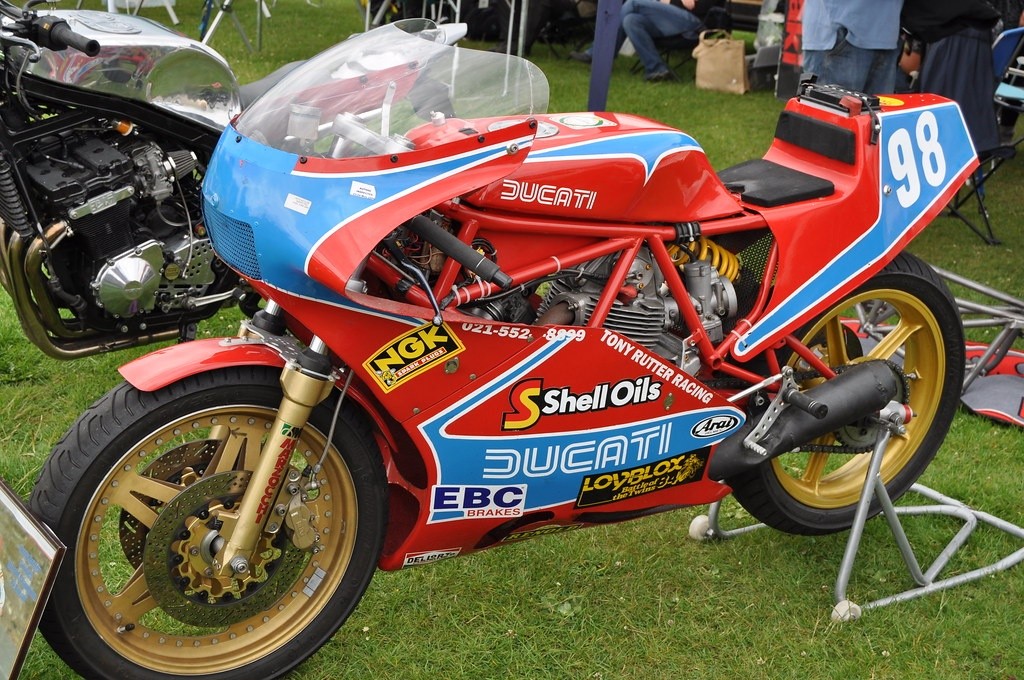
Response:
[23,13,980,680]
[0,0,473,367]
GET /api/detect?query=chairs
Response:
[628,0,734,84]
[956,26,1024,215]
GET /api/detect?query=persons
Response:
[797,0,903,105]
[569,0,724,84]
[917,25,1000,161]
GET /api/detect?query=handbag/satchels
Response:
[691,27,751,96]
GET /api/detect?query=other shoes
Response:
[646,72,675,83]
[570,50,592,64]
